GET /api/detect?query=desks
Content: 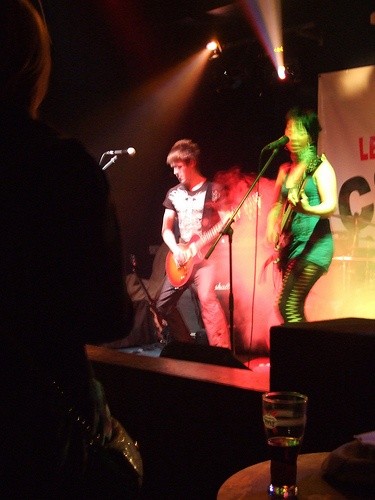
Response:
[217,451,345,500]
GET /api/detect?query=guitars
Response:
[164,190,260,289]
[274,156,321,252]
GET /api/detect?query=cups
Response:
[264,393,308,498]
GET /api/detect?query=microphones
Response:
[105,147,136,157]
[266,136,289,150]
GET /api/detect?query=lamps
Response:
[207,40,222,59]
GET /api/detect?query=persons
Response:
[155,139,233,348]
[0,0,134,500]
[266,110,338,324]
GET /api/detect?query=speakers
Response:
[159,341,249,370]
[267,316,375,454]
